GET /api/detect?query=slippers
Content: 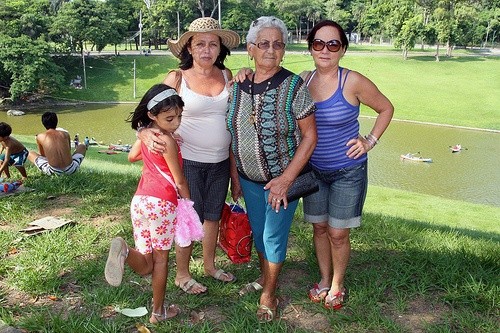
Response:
[214,269,234,281]
[324,288,346,310]
[257,299,279,323]
[182,279,207,295]
[248,278,279,291]
[308,283,331,303]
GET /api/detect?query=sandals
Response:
[105,237,129,287]
[150,305,181,324]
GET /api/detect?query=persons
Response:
[229,20,394,310]
[73,135,79,148]
[136,17,237,295]
[28,112,87,176]
[0,122,29,180]
[105,84,190,325]
[84,137,89,150]
[227,16,318,322]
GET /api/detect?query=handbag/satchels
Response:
[175,198,204,247]
[280,171,320,206]
[218,199,253,264]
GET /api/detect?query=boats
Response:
[7,109,25,116]
[448,144,462,152]
[401,154,432,163]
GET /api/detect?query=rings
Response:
[273,198,276,201]
[151,141,154,147]
[277,200,281,203]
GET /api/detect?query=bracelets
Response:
[364,133,378,146]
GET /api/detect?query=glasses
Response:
[312,39,343,52]
[251,41,286,50]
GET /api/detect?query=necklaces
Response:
[249,73,272,124]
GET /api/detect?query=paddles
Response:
[460,149,468,150]
[449,145,453,149]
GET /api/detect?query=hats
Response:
[167,17,240,59]
